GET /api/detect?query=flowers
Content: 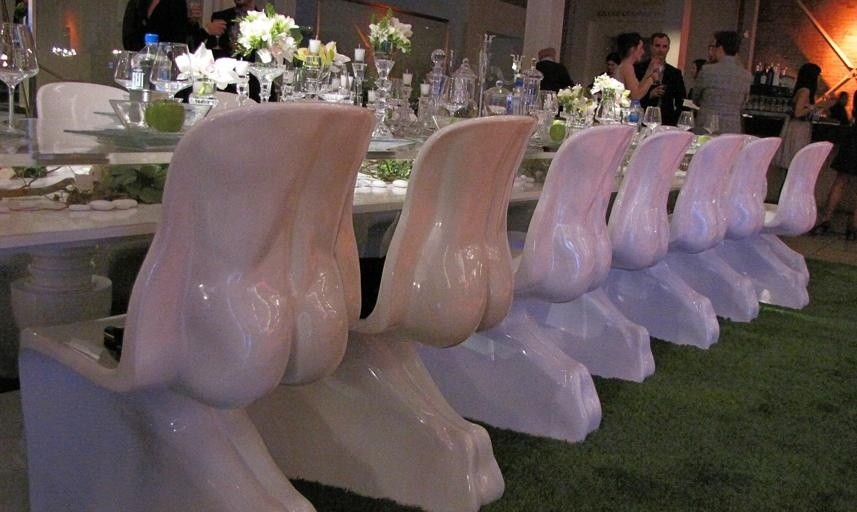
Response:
[364,7,414,59]
[230,4,306,65]
[294,40,350,74]
[176,43,238,92]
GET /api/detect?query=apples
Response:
[549,124,565,142]
[145,99,185,132]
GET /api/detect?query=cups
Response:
[112,42,195,98]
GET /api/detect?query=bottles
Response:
[131,33,172,102]
[750,60,796,93]
[504,77,526,114]
[628,100,642,124]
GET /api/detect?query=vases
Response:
[374,42,393,60]
[256,49,276,67]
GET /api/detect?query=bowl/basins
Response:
[107,96,213,128]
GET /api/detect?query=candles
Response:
[309,33,321,56]
[403,67,414,88]
[354,42,366,63]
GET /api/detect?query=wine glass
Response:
[644,107,721,138]
[439,74,468,123]
[0,24,37,129]
[533,88,617,133]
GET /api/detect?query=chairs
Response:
[663,131,747,343]
[17,102,476,511]
[2,84,709,333]
[504,124,641,435]
[722,137,802,317]
[759,139,836,300]
[596,130,712,381]
[350,113,589,502]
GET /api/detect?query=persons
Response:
[774,63,829,208]
[811,90,857,240]
[123,0,295,104]
[585,30,686,127]
[687,31,752,137]
[536,47,574,120]
